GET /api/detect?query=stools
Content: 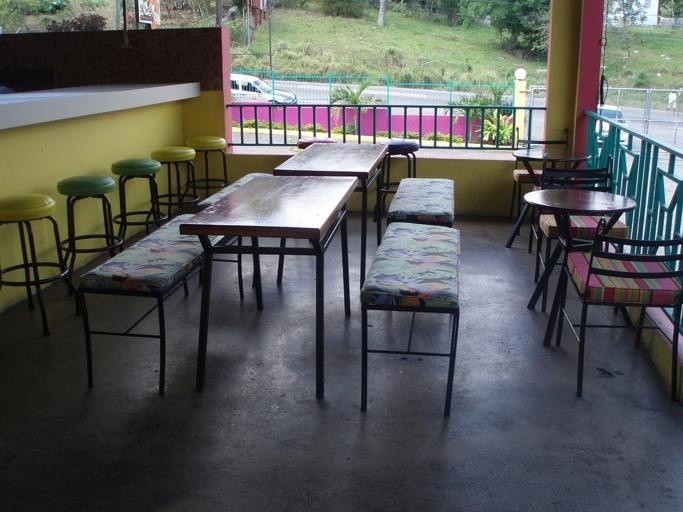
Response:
[298,138,337,149]
[113,158,168,245]
[0,193,68,346]
[150,146,201,226]
[185,134,230,197]
[373,140,419,223]
[55,175,116,296]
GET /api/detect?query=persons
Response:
[667,87,678,113]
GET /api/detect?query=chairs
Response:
[528,154,613,253]
[509,127,569,221]
[557,217,682,401]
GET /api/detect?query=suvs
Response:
[229,73,297,107]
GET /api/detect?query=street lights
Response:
[512,68,527,146]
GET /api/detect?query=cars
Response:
[594,103,631,147]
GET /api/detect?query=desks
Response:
[179,174,359,399]
[272,142,392,296]
[524,188,636,325]
[505,149,605,248]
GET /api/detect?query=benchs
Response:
[361,222,458,419]
[387,175,456,221]
[82,212,249,398]
[198,173,272,210]
